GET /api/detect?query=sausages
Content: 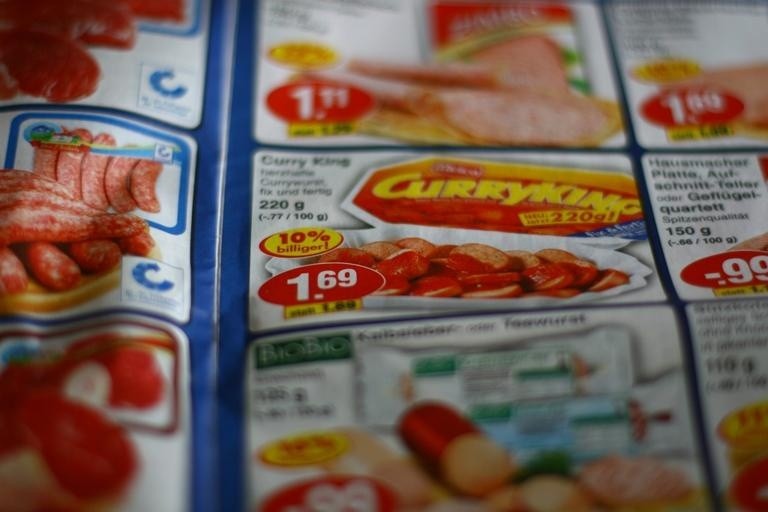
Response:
[0,128,162,297]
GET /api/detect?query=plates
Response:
[264,224,652,313]
